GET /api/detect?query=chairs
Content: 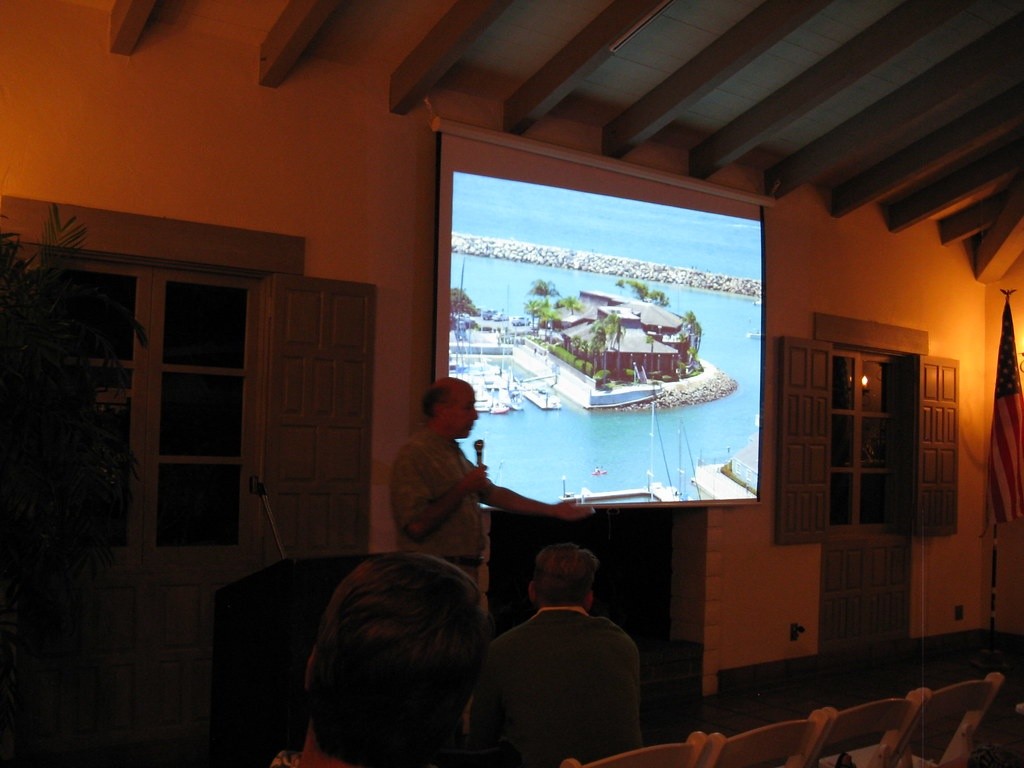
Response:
[560,671,1006,768]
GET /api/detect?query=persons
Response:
[269,548,495,768]
[470,541,644,768]
[388,378,597,604]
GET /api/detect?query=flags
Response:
[977,302,1024,539]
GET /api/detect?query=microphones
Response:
[475,440,484,497]
